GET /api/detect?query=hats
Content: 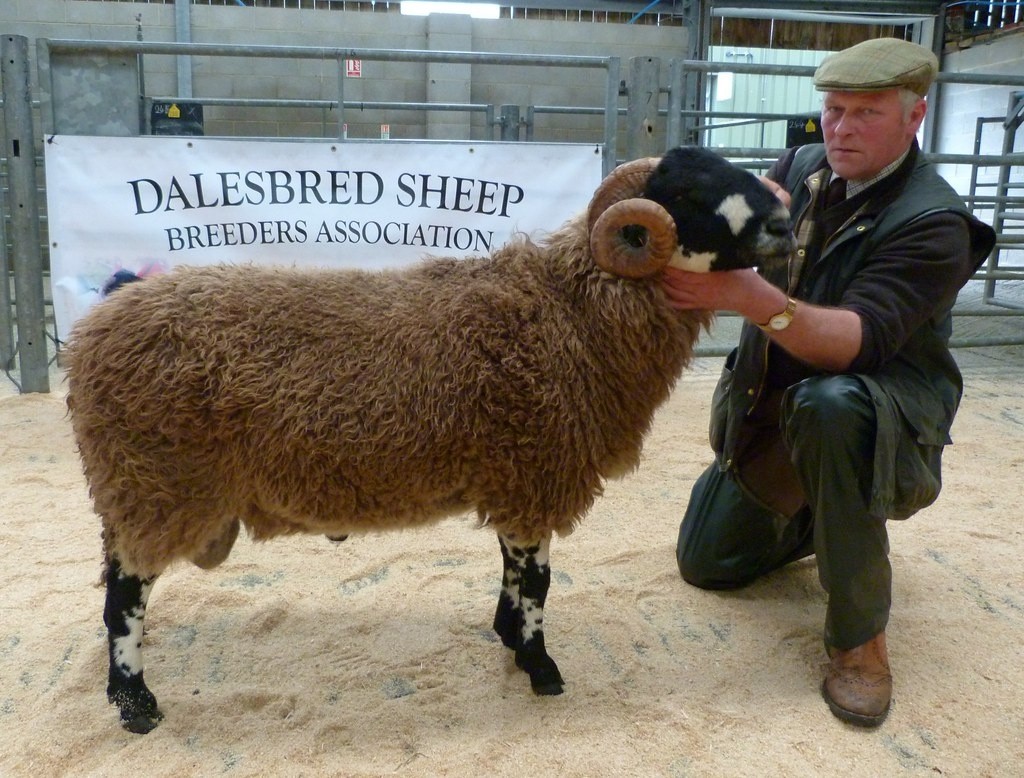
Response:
[813,38,937,100]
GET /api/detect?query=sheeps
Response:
[57,144,799,734]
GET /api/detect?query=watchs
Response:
[754,297,796,333]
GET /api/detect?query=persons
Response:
[656,37,996,726]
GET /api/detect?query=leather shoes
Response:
[823,627,893,727]
